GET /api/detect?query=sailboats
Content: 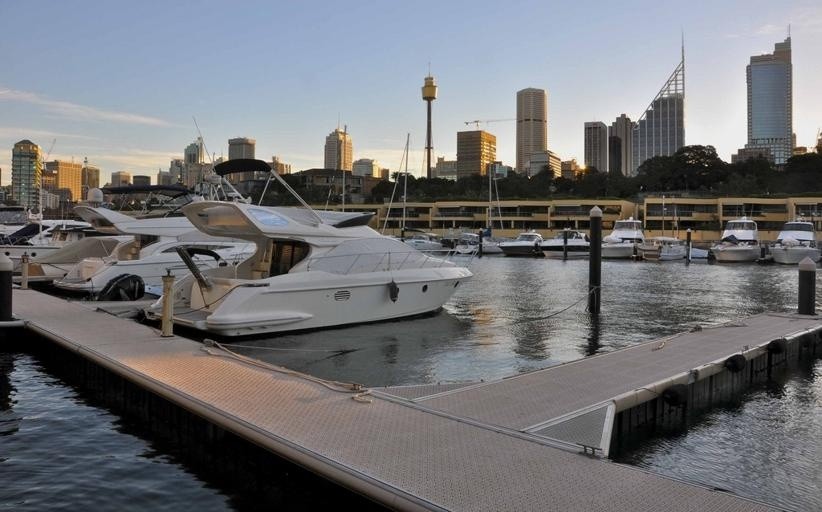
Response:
[455,141,506,255]
[380,133,443,250]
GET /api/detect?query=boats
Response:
[0,158,266,301]
[709,216,762,263]
[636,194,686,262]
[539,226,590,258]
[769,212,822,265]
[601,217,646,261]
[141,199,474,342]
[496,226,544,257]
